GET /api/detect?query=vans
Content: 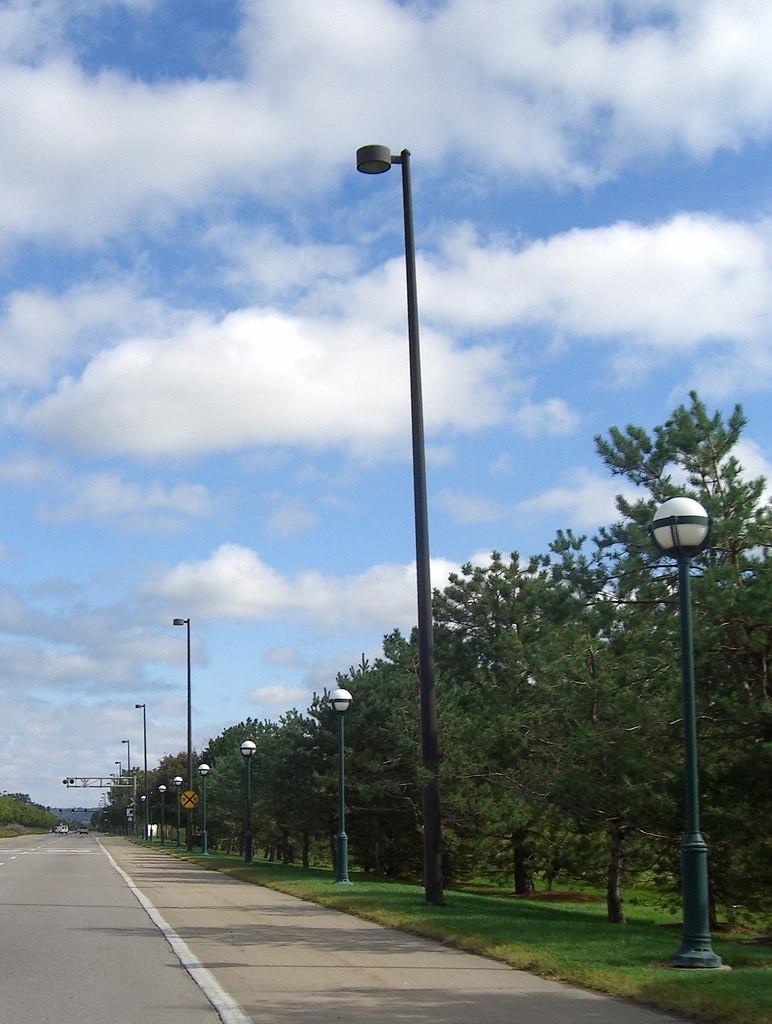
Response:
[54,825,68,834]
[80,826,88,834]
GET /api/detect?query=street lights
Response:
[123,740,132,836]
[355,143,447,908]
[158,785,166,845]
[172,617,195,854]
[240,740,257,864]
[136,704,148,842]
[141,796,146,841]
[198,763,210,857]
[327,687,355,887]
[649,495,722,970]
[174,776,183,847]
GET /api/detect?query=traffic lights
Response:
[84,809,87,814]
[59,809,62,814]
[71,809,74,814]
